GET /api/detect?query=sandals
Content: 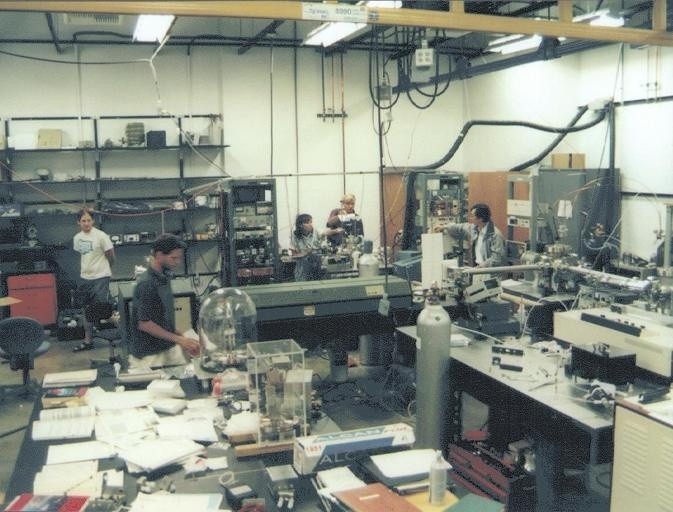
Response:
[73,343,92,351]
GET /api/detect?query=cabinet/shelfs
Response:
[4,114,228,287]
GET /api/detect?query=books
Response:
[2,368,223,512]
[314,446,504,512]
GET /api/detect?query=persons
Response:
[433,202,509,281]
[72,208,120,352]
[130,232,200,360]
[326,194,364,264]
[288,213,345,279]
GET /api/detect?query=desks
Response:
[2,374,345,512]
[393,318,661,511]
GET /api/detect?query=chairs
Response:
[0,317,50,394]
[86,301,122,363]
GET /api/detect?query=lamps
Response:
[300,0,404,51]
[484,8,625,56]
